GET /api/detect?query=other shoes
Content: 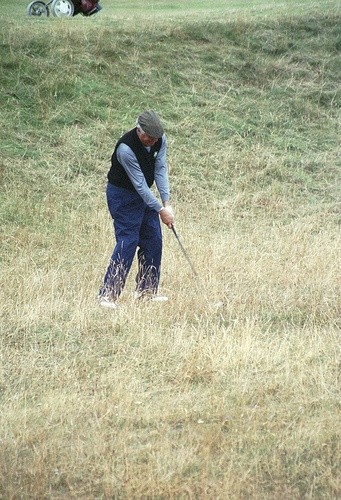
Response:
[150,296,169,301]
[100,299,116,309]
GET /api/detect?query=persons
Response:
[98,110,175,310]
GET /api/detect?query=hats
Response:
[138,110,164,139]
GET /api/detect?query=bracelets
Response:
[157,206,165,212]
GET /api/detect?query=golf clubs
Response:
[171,225,197,276]
